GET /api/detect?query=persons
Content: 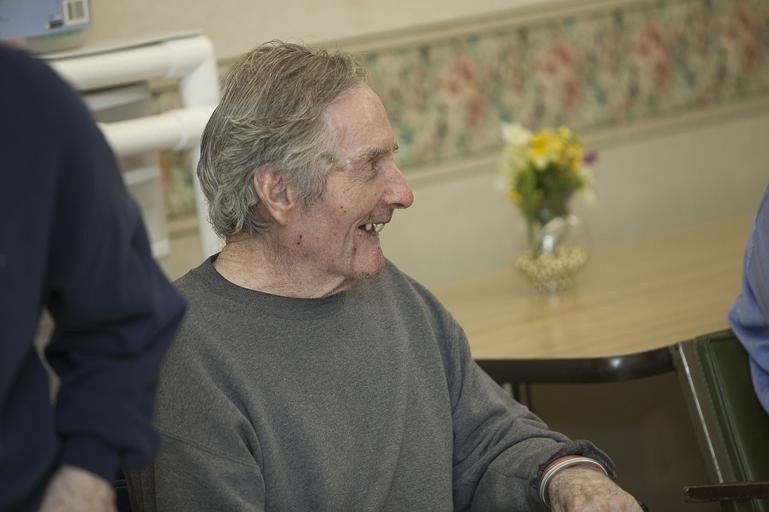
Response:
[728,189,769,416]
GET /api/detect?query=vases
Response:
[518,237,594,295]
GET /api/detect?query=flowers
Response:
[503,128,594,236]
